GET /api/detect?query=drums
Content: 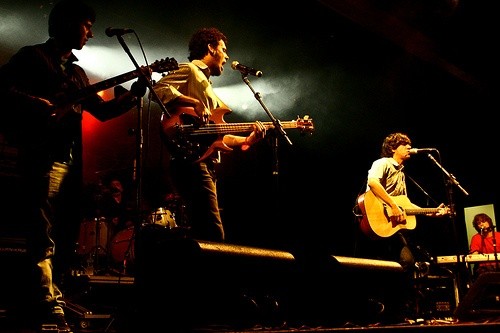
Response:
[76,216,114,256]
[108,217,137,273]
[140,207,178,231]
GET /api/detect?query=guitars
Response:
[33,58,179,117]
[159,107,315,162]
[355,190,453,239]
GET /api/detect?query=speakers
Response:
[306,255,404,324]
[154,238,295,325]
[452,272,500,318]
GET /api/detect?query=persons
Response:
[353,132,451,323]
[0,0,152,333]
[148,27,267,252]
[260,144,338,274]
[469,213,500,283]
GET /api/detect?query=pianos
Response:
[434,253,500,316]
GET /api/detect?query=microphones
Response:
[231,60,263,78]
[104,26,133,38]
[409,147,435,154]
[481,226,485,229]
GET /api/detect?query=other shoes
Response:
[40,298,71,332]
[382,317,403,324]
[401,308,425,324]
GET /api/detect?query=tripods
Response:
[87,216,107,269]
